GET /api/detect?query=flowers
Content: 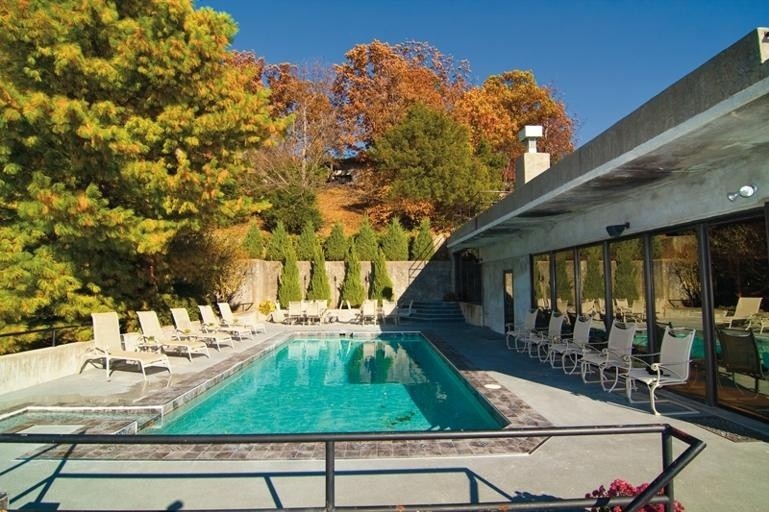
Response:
[582,479,686,512]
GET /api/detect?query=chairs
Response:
[538,297,661,324]
[714,298,769,401]
[78,302,266,381]
[266,298,414,328]
[504,309,701,417]
